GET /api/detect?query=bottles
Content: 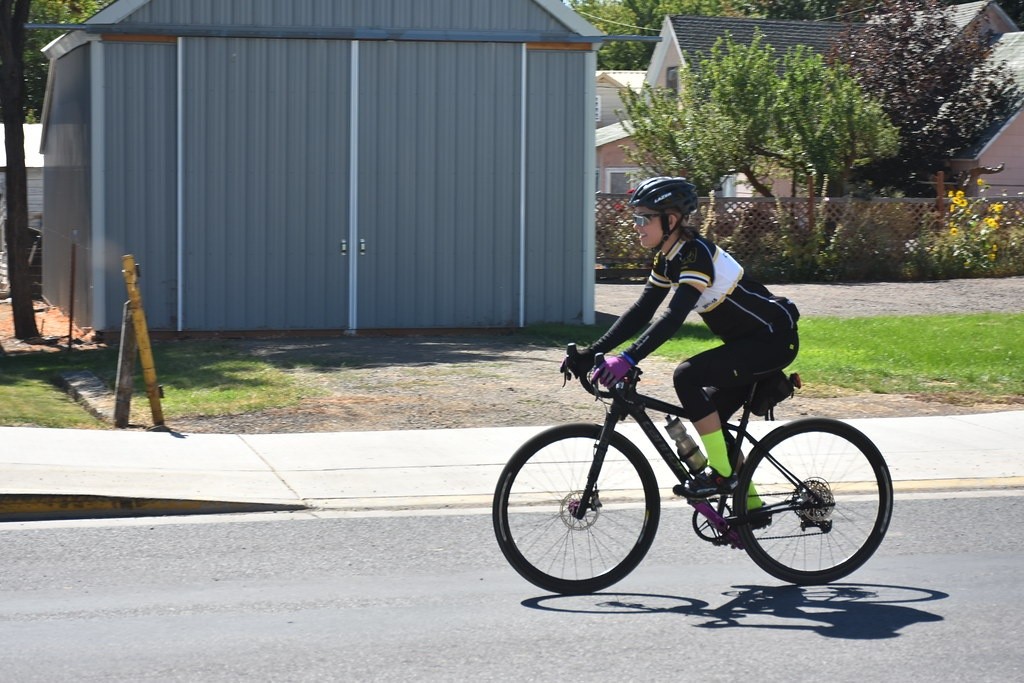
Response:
[664,414,708,464]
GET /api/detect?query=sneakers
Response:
[749,502,772,529]
[673,465,739,500]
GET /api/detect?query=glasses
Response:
[632,212,674,227]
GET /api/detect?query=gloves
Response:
[591,354,633,389]
[560,349,595,371]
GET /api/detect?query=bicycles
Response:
[490,339,896,596]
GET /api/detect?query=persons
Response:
[560,177,802,531]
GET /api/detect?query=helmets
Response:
[627,176,699,222]
